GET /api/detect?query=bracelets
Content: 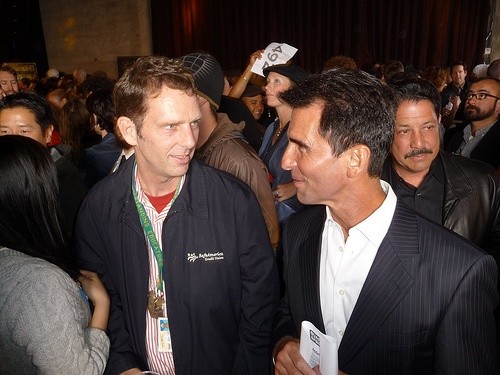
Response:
[241,74,250,82]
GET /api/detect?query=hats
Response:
[263,64,306,84]
[242,85,265,97]
[176,53,221,108]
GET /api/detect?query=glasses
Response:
[463,93,500,100]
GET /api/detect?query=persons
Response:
[0,48,500,260]
[269,65,500,375]
[71,54,282,375]
[0,133,112,375]
